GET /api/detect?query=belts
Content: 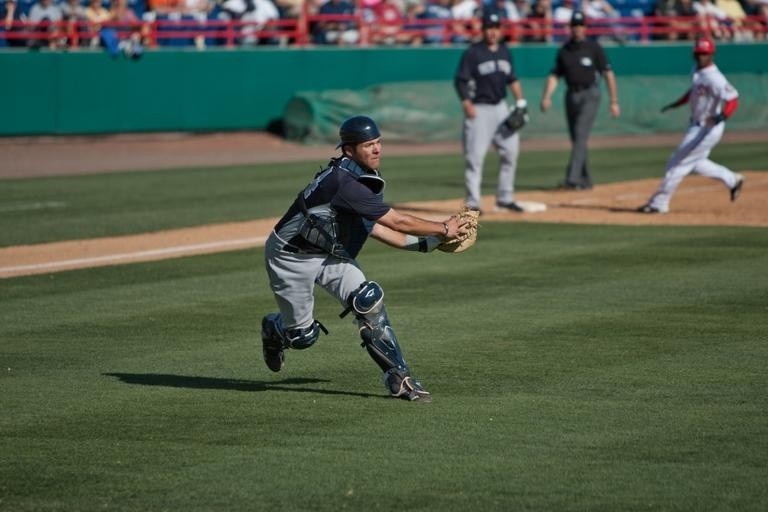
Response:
[690,119,699,125]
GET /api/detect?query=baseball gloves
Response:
[498,107,529,137]
[438,209,480,252]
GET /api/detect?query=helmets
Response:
[571,11,585,27]
[335,117,380,151]
[693,37,714,54]
[481,14,500,29]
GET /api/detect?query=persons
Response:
[454,14,528,216]
[261,116,468,402]
[634,37,744,213]
[540,12,618,192]
[0,0,768,62]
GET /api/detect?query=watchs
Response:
[442,223,449,236]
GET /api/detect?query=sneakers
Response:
[262,313,285,372]
[389,373,432,403]
[639,205,657,213]
[495,202,523,212]
[730,178,744,200]
